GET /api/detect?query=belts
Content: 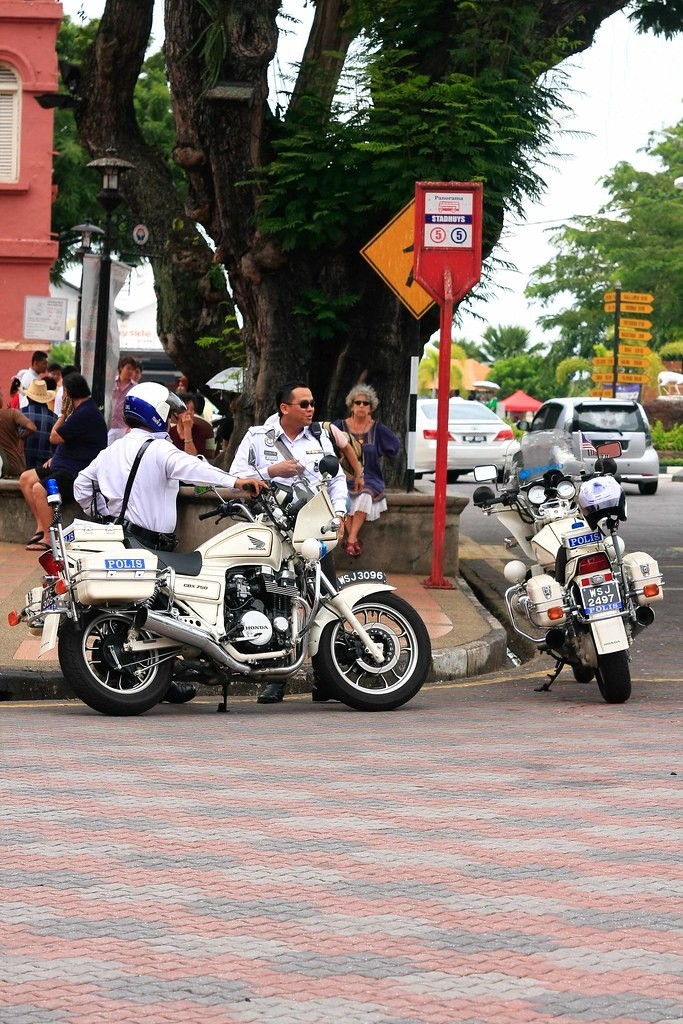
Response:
[108,513,164,545]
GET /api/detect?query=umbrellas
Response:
[204,367,245,393]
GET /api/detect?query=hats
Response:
[16,378,56,403]
[486,391,493,394]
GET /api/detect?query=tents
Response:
[500,390,544,421]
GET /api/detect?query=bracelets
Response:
[184,439,192,442]
[58,414,65,419]
[355,473,364,478]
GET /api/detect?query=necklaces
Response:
[352,419,368,444]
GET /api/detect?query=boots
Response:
[256,679,284,704]
[311,652,334,702]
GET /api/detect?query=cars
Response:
[511,397,659,495]
[415,397,522,484]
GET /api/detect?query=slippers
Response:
[26,532,53,550]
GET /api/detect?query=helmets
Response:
[123,380,171,432]
[577,475,628,531]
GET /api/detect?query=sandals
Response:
[345,535,364,560]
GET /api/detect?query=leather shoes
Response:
[160,683,197,705]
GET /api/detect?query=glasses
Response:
[351,399,373,407]
[283,399,317,409]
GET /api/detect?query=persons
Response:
[106,356,238,460]
[71,381,269,706]
[0,351,82,475]
[229,381,349,703]
[331,383,399,557]
[481,390,497,414]
[20,373,108,551]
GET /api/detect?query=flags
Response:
[582,434,597,455]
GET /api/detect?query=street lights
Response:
[86,146,135,420]
[70,216,105,373]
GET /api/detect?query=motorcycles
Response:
[8,441,431,716]
[473,430,664,704]
